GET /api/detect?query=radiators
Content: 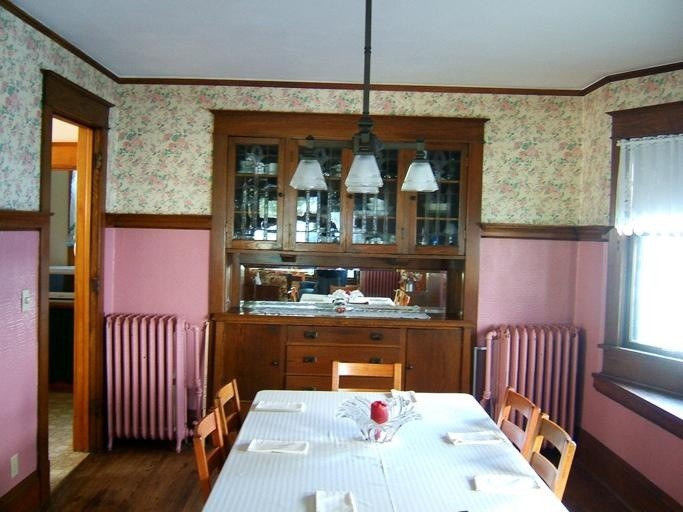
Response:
[105,311,197,452]
[485,322,584,446]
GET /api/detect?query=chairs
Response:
[329,357,404,391]
[191,399,225,507]
[495,382,541,459]
[215,378,242,445]
[527,411,578,501]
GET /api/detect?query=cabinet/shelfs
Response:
[205,105,491,421]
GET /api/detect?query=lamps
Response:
[285,0,439,198]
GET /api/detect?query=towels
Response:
[445,430,500,447]
[314,490,355,511]
[471,474,539,494]
[247,439,307,456]
[254,401,305,413]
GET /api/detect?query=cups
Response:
[237,152,455,250]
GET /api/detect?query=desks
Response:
[198,387,567,512]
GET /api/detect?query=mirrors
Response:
[49,168,75,267]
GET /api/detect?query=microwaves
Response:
[47,266,77,298]
